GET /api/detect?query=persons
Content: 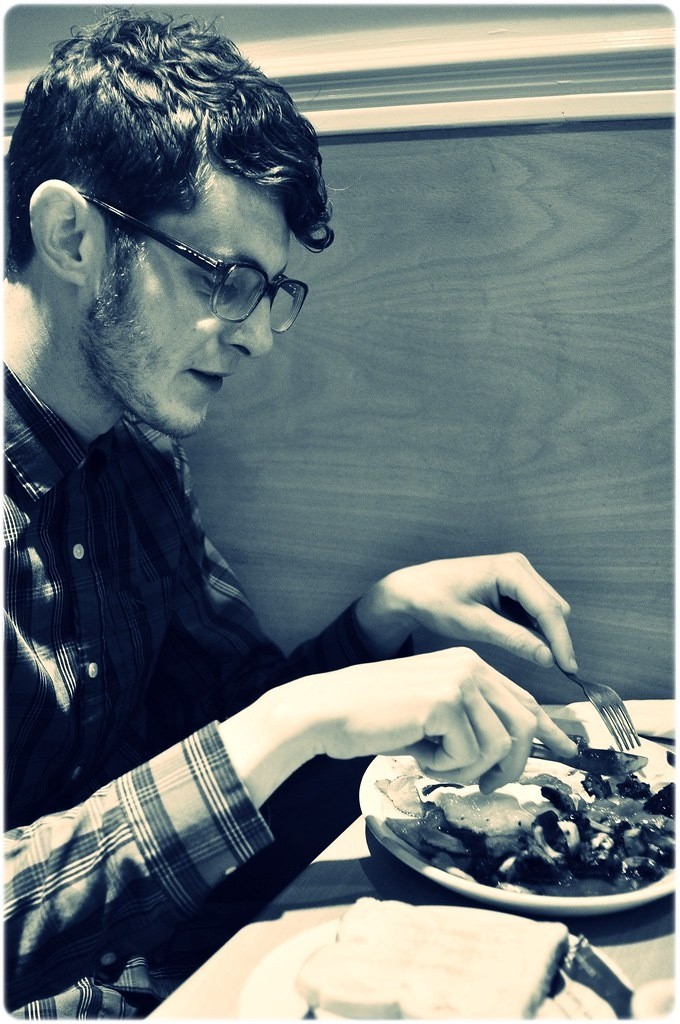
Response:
[5,13,581,1019]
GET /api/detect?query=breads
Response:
[296,898,568,1020]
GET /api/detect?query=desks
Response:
[141,697,675,1021]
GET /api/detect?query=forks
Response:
[526,609,642,752]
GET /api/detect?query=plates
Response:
[359,731,676,918]
[236,902,632,1021]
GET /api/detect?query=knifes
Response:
[424,731,649,777]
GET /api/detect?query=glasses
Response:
[76,190,310,335]
